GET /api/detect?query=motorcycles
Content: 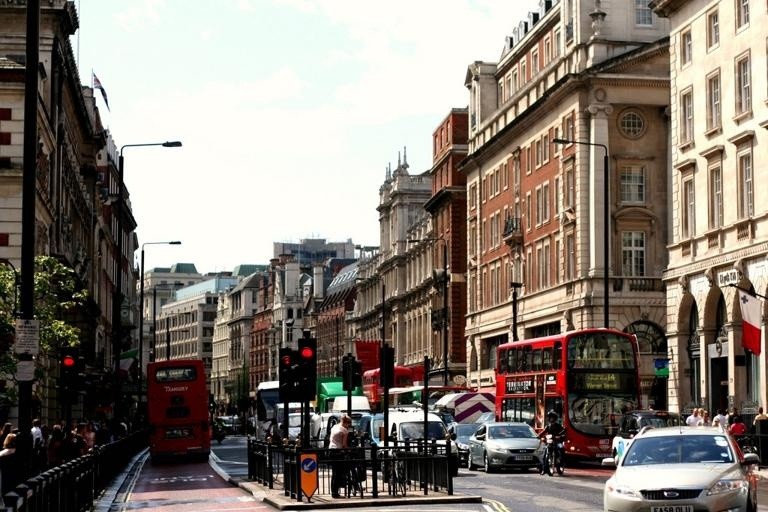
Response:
[215,424,226,444]
[536,430,566,475]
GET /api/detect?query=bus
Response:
[252,380,313,441]
[363,366,413,410]
[413,386,476,406]
[147,358,215,465]
[492,327,644,468]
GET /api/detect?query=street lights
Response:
[408,237,448,384]
[111,141,184,438]
[549,136,611,331]
[151,281,191,364]
[138,241,182,428]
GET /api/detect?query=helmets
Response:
[545,410,559,423]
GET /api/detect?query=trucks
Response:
[316,376,363,414]
[428,392,496,424]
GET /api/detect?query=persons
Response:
[1,418,132,482]
[753,407,767,426]
[686,406,746,447]
[329,416,352,498]
[538,411,566,475]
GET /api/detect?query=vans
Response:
[332,395,372,414]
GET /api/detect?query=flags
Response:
[739,289,762,356]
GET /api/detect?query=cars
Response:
[274,403,544,475]
[217,416,242,433]
[601,410,759,512]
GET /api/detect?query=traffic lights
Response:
[297,338,317,402]
[344,355,362,392]
[278,348,300,403]
[58,347,80,405]
